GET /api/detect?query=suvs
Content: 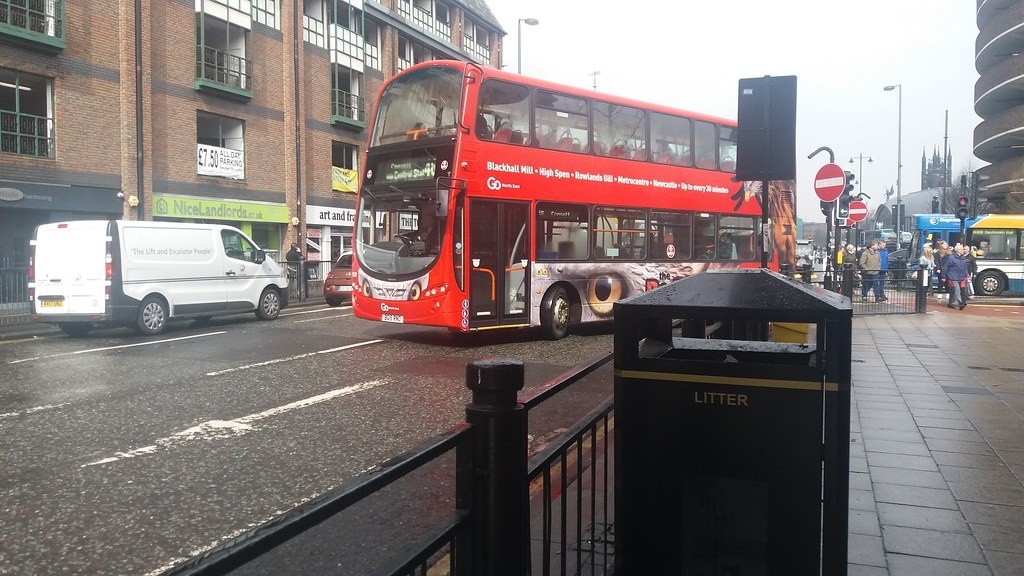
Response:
[899,232,912,248]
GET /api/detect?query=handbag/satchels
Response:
[966,278,975,299]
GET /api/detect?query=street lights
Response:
[517,18,539,76]
[884,84,903,250]
[849,153,876,193]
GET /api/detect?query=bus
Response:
[912,213,1024,298]
[859,227,898,250]
[349,58,797,338]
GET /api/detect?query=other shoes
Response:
[937,294,942,298]
[949,304,956,309]
[862,296,867,302]
[876,296,885,302]
[960,302,967,310]
[946,292,950,299]
[881,294,888,300]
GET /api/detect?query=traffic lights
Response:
[838,170,855,219]
[955,194,970,218]
[819,200,833,216]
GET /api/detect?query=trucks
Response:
[795,240,816,273]
[28,219,290,338]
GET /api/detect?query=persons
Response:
[877,241,888,300]
[860,240,885,301]
[656,140,672,155]
[407,122,430,140]
[941,243,970,310]
[720,232,738,260]
[948,274,972,307]
[286,244,303,298]
[476,108,487,139]
[920,240,953,299]
[978,241,988,256]
[857,244,867,269]
[399,213,436,249]
[832,244,855,297]
[963,244,977,300]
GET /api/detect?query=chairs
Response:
[557,241,575,259]
[592,242,630,259]
[482,121,734,173]
[427,130,452,138]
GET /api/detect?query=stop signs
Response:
[814,164,846,202]
[848,201,868,222]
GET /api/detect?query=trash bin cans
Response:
[613,268,853,576]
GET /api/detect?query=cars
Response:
[323,251,353,307]
[885,249,911,283]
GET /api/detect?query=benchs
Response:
[698,236,754,259]
